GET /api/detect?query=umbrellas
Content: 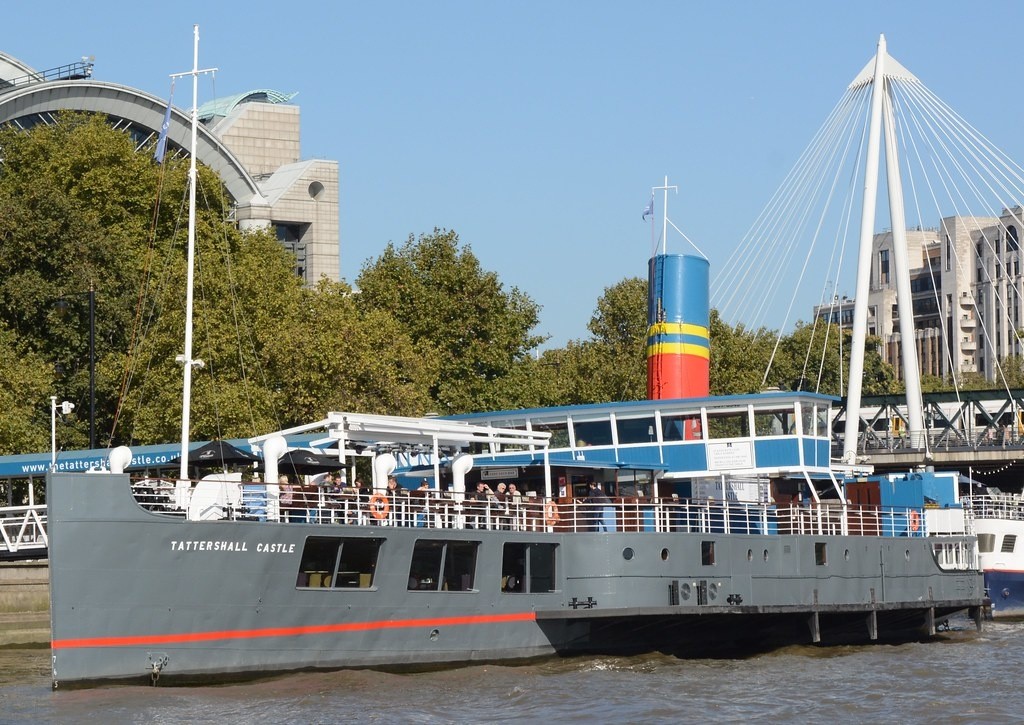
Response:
[166,434,264,474]
[250,447,353,484]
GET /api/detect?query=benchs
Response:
[279,487,543,532]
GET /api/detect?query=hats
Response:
[421,477,430,484]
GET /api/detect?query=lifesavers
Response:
[369,493,389,519]
[545,501,558,525]
[911,510,919,531]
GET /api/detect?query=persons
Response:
[987,426,995,446]
[1003,424,1011,446]
[582,481,608,532]
[412,481,429,528]
[243,474,293,523]
[318,473,371,526]
[385,479,408,526]
[465,482,522,531]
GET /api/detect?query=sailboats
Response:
[46,21,1021,690]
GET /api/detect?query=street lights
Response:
[49,395,76,472]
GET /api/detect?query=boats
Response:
[960,494,1024,615]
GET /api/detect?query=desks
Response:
[333,497,355,524]
